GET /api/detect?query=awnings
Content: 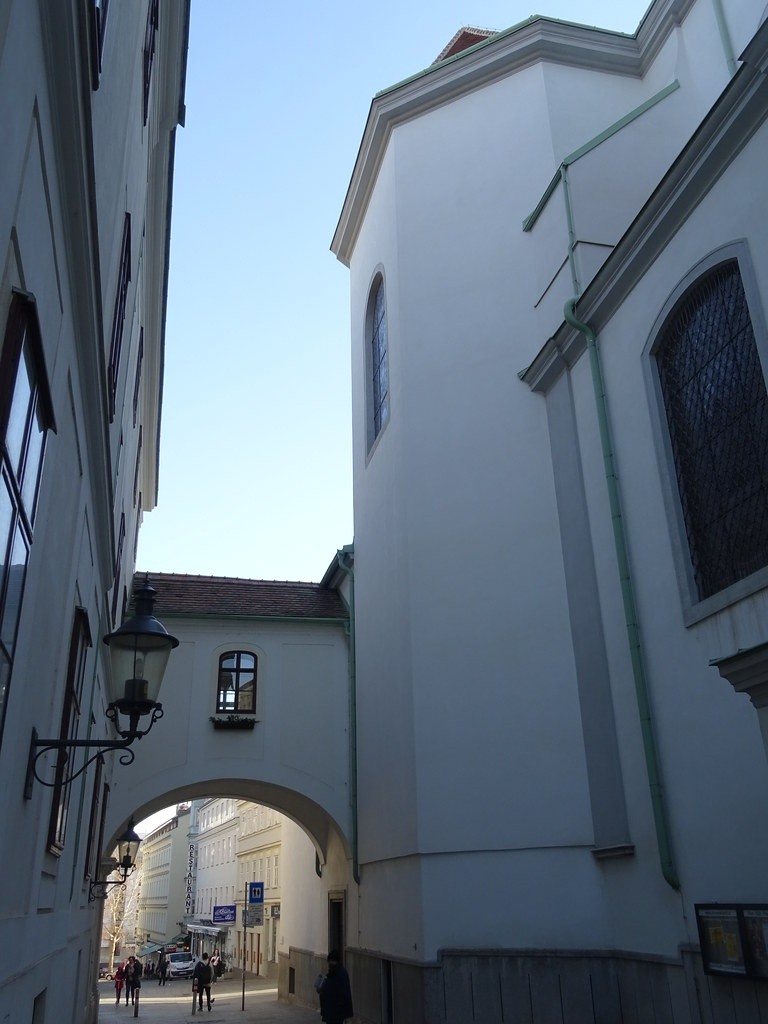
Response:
[136,945,163,957]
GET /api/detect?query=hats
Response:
[327,949,338,962]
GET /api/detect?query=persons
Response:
[135,957,168,987]
[114,956,142,1007]
[192,949,222,1012]
[313,948,354,1024]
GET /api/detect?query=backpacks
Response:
[198,960,211,989]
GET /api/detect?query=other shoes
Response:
[125,1003,128,1006]
[132,1001,134,1005]
[207,1003,211,1012]
[198,1005,202,1011]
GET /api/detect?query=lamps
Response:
[23,573,179,801]
[88,814,143,903]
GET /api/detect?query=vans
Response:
[159,952,195,981]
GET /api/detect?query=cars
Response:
[99,967,118,981]
